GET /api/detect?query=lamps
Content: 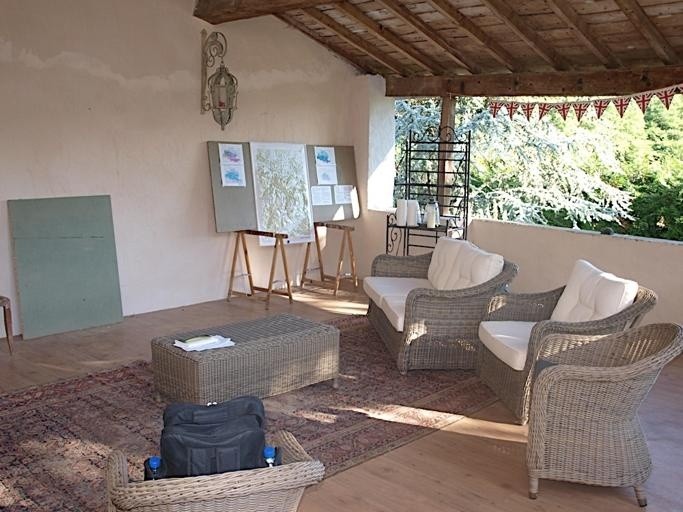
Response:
[195,25,240,138]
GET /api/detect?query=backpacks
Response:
[144,395,282,480]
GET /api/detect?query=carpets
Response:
[0,308,501,512]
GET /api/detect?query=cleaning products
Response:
[422,198,440,225]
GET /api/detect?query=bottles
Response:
[423,199,440,226]
[148,457,160,480]
[264,446,277,468]
[427,202,435,228]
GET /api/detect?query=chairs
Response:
[468,257,659,427]
[99,424,330,512]
[523,319,682,510]
[359,232,520,379]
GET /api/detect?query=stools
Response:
[0,294,14,358]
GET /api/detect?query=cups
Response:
[397,199,420,228]
